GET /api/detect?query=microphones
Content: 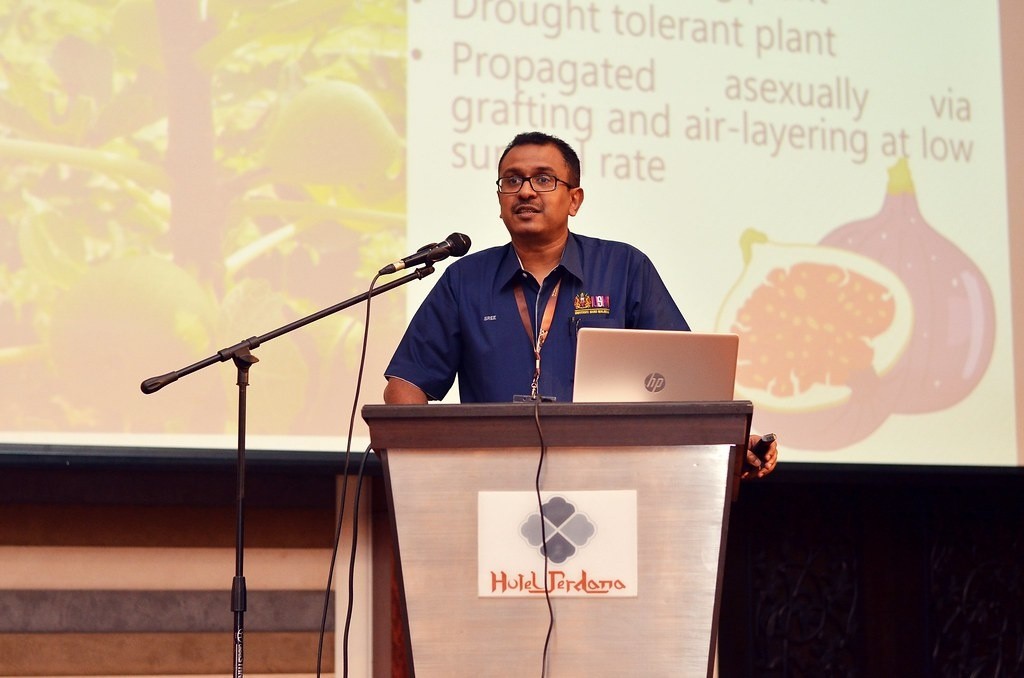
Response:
[379,233,472,275]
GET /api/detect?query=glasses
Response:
[496,174,575,193]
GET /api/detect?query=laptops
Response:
[572,327,739,404]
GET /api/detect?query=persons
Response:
[383,132,779,480]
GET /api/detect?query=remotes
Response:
[747,434,777,473]
